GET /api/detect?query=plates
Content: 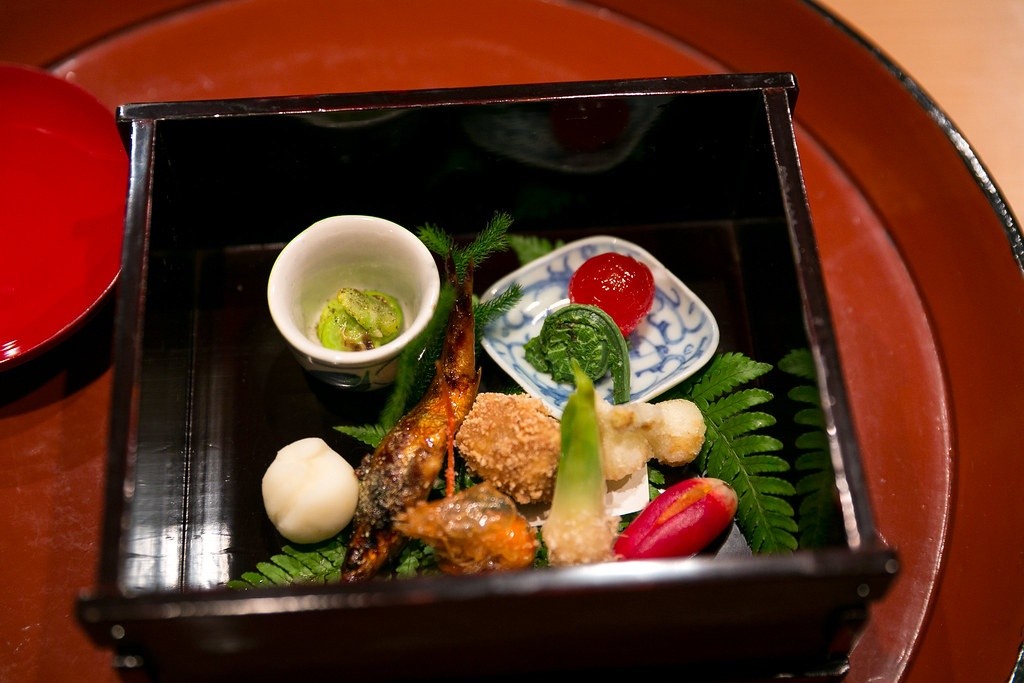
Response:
[0,62,128,370]
[477,236,720,419]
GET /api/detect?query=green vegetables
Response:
[224,469,478,586]
[780,347,842,559]
[667,352,798,559]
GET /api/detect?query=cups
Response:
[268,213,440,394]
